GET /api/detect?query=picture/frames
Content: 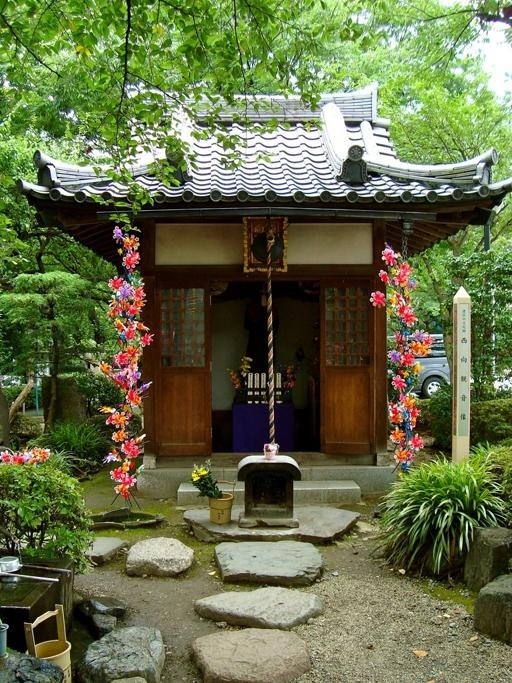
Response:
[241,215,290,274]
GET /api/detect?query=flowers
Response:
[224,367,242,392]
[369,241,435,471]
[240,353,254,389]
[280,360,304,396]
[187,461,225,498]
[97,224,156,501]
[0,446,97,579]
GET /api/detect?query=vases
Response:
[0,622,9,657]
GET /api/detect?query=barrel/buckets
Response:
[209,491,233,524]
[24,604,72,683]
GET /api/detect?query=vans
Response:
[383,331,451,396]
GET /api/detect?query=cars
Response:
[1,374,40,386]
[493,375,511,390]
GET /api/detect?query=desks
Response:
[232,399,295,453]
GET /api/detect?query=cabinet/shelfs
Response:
[0,557,76,654]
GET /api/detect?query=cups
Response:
[260,444,279,458]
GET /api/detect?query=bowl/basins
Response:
[1,556,23,572]
